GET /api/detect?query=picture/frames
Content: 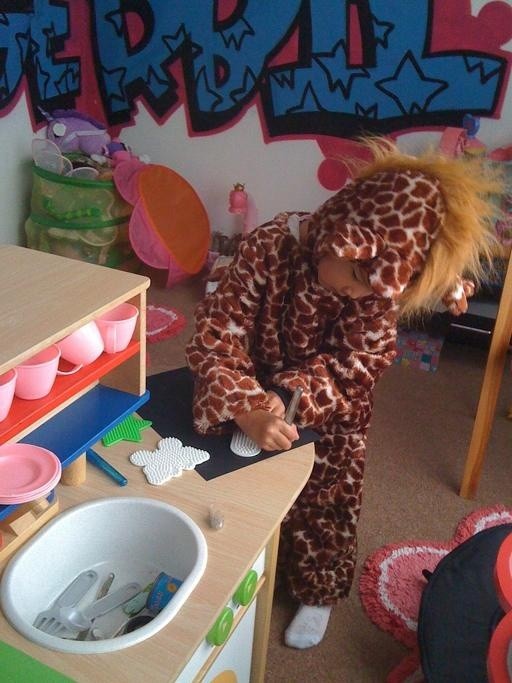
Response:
[416,524,511,683]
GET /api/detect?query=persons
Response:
[186,135,504,651]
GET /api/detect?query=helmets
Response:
[0,443,62,504]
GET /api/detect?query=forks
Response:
[33,569,98,635]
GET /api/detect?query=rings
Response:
[283,385,304,425]
[86,449,127,487]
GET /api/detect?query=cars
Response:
[14,344,62,401]
[96,302,140,355]
[55,319,105,377]
[0,367,18,423]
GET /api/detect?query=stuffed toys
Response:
[46,110,111,158]
[104,137,131,168]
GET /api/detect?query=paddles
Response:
[145,300,186,343]
[357,505,511,683]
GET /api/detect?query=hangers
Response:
[64,582,142,633]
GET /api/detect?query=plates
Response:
[1,242,316,683]
